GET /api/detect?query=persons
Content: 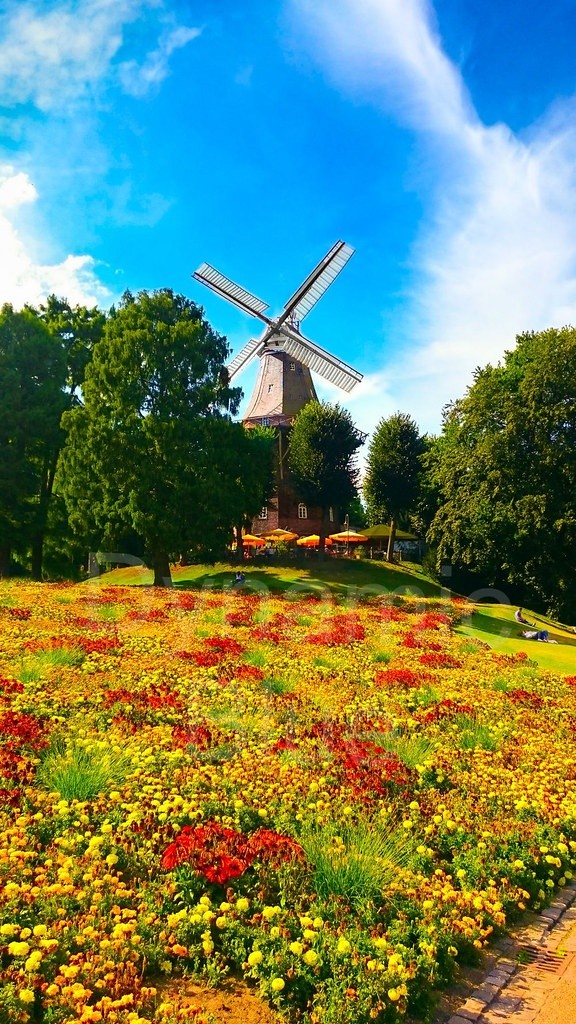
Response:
[235,571,242,584]
[516,608,536,627]
[519,629,548,642]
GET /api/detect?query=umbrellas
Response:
[231,525,418,556]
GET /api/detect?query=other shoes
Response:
[532,623,536,627]
